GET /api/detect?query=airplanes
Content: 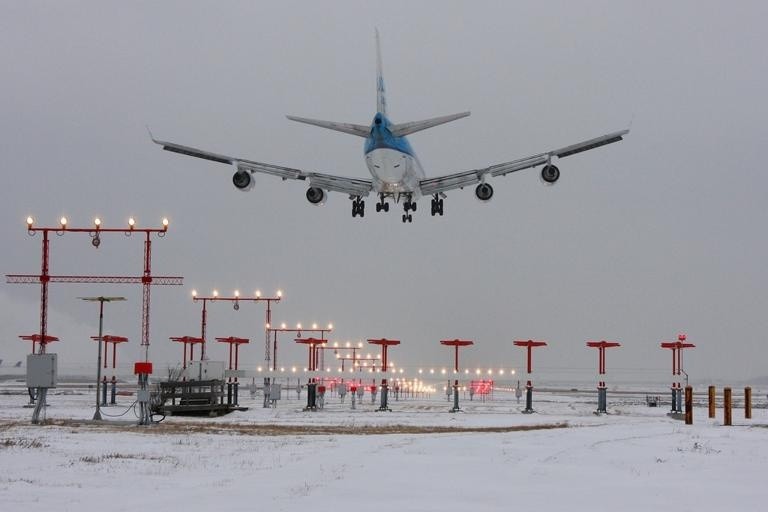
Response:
[144,26,631,223]
